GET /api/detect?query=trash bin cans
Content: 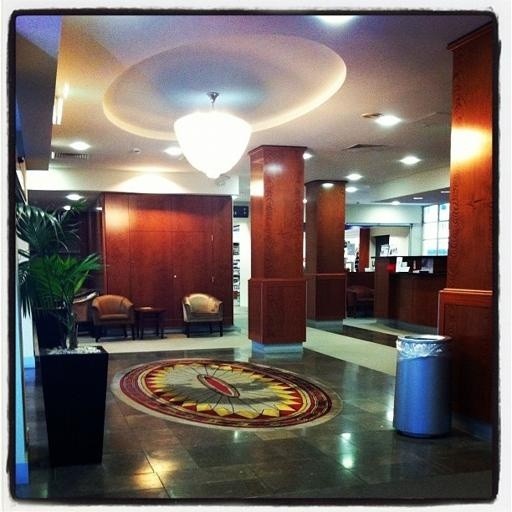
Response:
[393,334,453,439]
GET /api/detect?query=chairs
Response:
[92,294,136,341]
[180,292,226,337]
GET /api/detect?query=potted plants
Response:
[16,197,110,468]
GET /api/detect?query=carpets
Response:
[112,356,343,435]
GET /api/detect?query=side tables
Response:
[134,305,169,339]
